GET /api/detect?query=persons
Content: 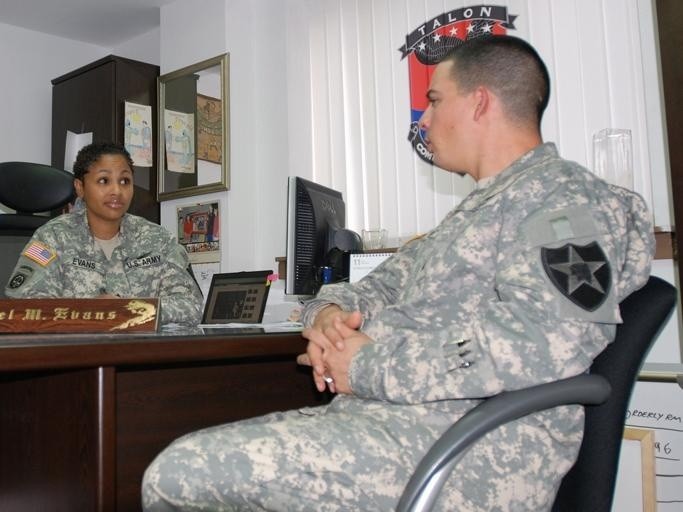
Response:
[4,143,204,326]
[142,35,658,512]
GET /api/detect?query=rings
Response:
[322,376,332,383]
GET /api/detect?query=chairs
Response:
[0,158,81,307]
[388,272,680,511]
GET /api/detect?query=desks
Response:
[0,306,334,512]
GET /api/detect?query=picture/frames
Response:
[608,423,660,512]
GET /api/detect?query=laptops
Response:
[198,269,274,326]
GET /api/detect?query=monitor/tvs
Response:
[286,178,359,296]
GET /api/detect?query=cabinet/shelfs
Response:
[45,51,160,229]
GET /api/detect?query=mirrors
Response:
[153,49,234,203]
